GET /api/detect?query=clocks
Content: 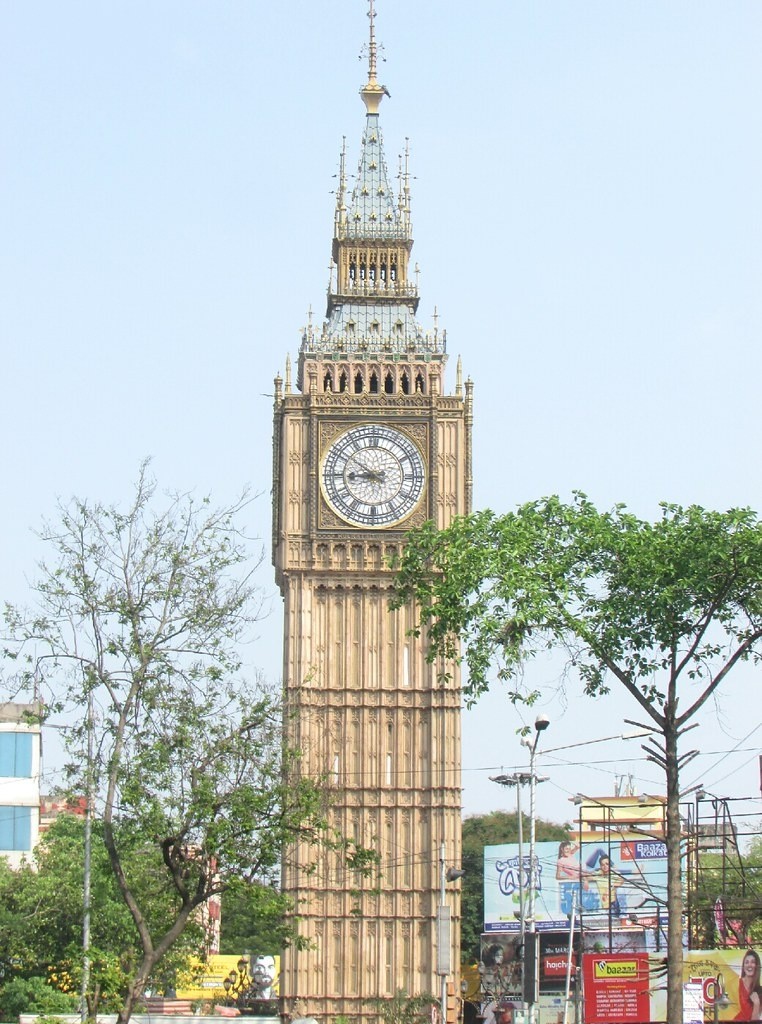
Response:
[320,421,429,530]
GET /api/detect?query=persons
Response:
[236,955,277,999]
[583,856,624,918]
[492,1001,517,1024]
[480,944,504,993]
[556,841,644,879]
[733,949,762,1024]
[515,1011,525,1024]
[505,944,525,995]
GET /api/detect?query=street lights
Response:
[519,712,655,1024]
